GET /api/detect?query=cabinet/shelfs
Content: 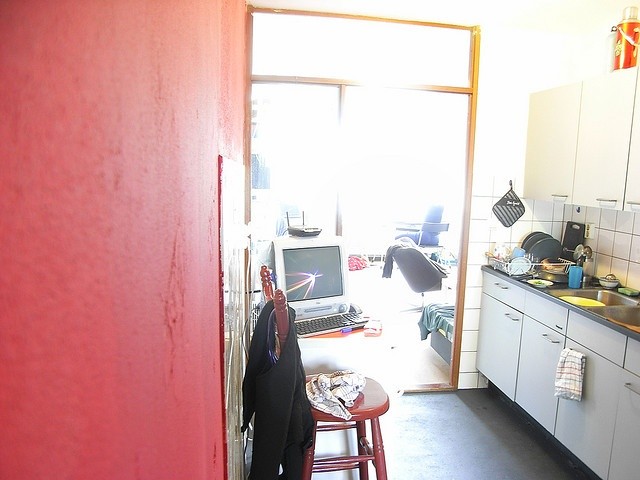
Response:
[608,337,640,480]
[523,82,582,204]
[623,66,640,213]
[572,67,638,211]
[515,288,566,435]
[554,310,621,480]
[475,270,523,402]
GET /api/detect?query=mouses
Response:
[350,304,363,315]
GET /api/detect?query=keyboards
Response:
[293,312,369,339]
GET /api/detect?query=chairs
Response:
[393,236,450,313]
[260,265,389,480]
[395,206,445,247]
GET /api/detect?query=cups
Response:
[567,266,583,289]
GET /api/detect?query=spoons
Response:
[584,245,593,261]
[572,244,584,262]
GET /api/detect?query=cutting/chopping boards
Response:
[561,220,585,262]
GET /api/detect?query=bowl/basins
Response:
[598,277,620,290]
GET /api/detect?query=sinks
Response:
[546,289,638,307]
[587,307,640,331]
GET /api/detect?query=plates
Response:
[526,279,554,288]
[523,233,554,249]
[507,257,531,275]
[521,231,542,248]
[529,238,561,260]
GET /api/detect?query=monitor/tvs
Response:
[274,235,350,323]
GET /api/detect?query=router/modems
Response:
[286,210,322,237]
